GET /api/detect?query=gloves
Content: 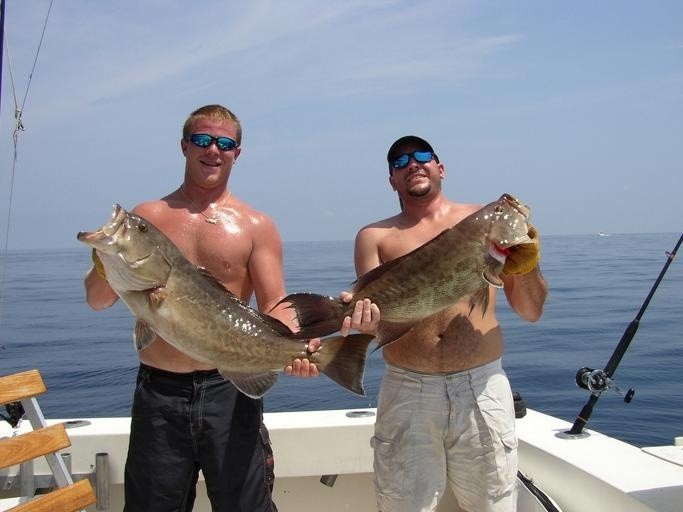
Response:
[504,225,539,276]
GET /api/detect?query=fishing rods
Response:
[570,233,683,434]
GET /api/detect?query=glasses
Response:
[392,151,435,167]
[187,134,239,150]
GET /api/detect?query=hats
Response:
[388,135,439,175]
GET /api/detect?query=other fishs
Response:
[266,193,541,356]
[76,202,376,401]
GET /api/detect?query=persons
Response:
[340,134,548,512]
[83,103,322,512]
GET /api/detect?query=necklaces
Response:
[177,184,231,224]
